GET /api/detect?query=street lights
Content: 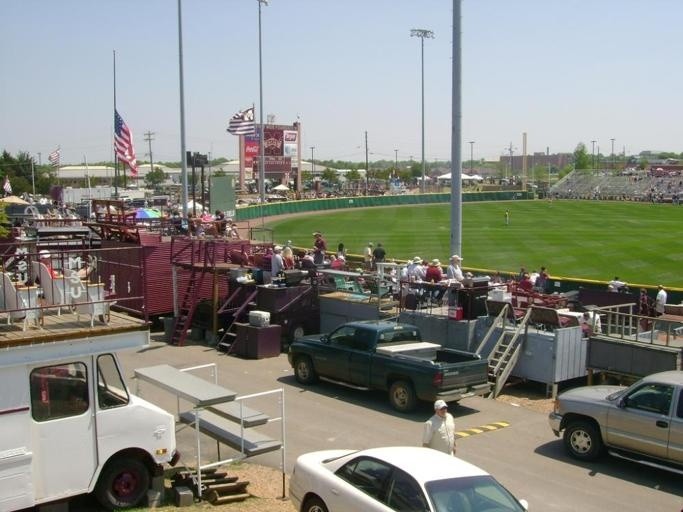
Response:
[309,147,315,173]
[409,26,436,191]
[256,0,270,206]
[468,141,475,175]
[591,140,596,169]
[394,150,399,168]
[611,138,615,169]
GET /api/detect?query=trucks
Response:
[0,242,179,512]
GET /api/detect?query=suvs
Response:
[548,370,683,481]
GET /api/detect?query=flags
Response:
[2,177,12,194]
[226,107,256,137]
[114,107,138,176]
[48,148,60,166]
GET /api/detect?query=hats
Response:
[412,257,424,264]
[434,399,449,412]
[273,245,284,253]
[429,258,442,267]
[449,255,464,262]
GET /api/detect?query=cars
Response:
[286,447,530,511]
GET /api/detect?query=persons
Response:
[630,168,683,206]
[607,277,632,294]
[37,250,97,280]
[639,287,651,331]
[519,266,550,290]
[652,284,668,315]
[504,209,511,224]
[173,209,225,239]
[576,311,602,337]
[270,230,465,283]
[422,398,457,459]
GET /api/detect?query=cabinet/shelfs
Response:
[129,362,285,463]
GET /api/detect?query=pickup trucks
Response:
[286,316,493,411]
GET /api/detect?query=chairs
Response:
[0,197,228,327]
